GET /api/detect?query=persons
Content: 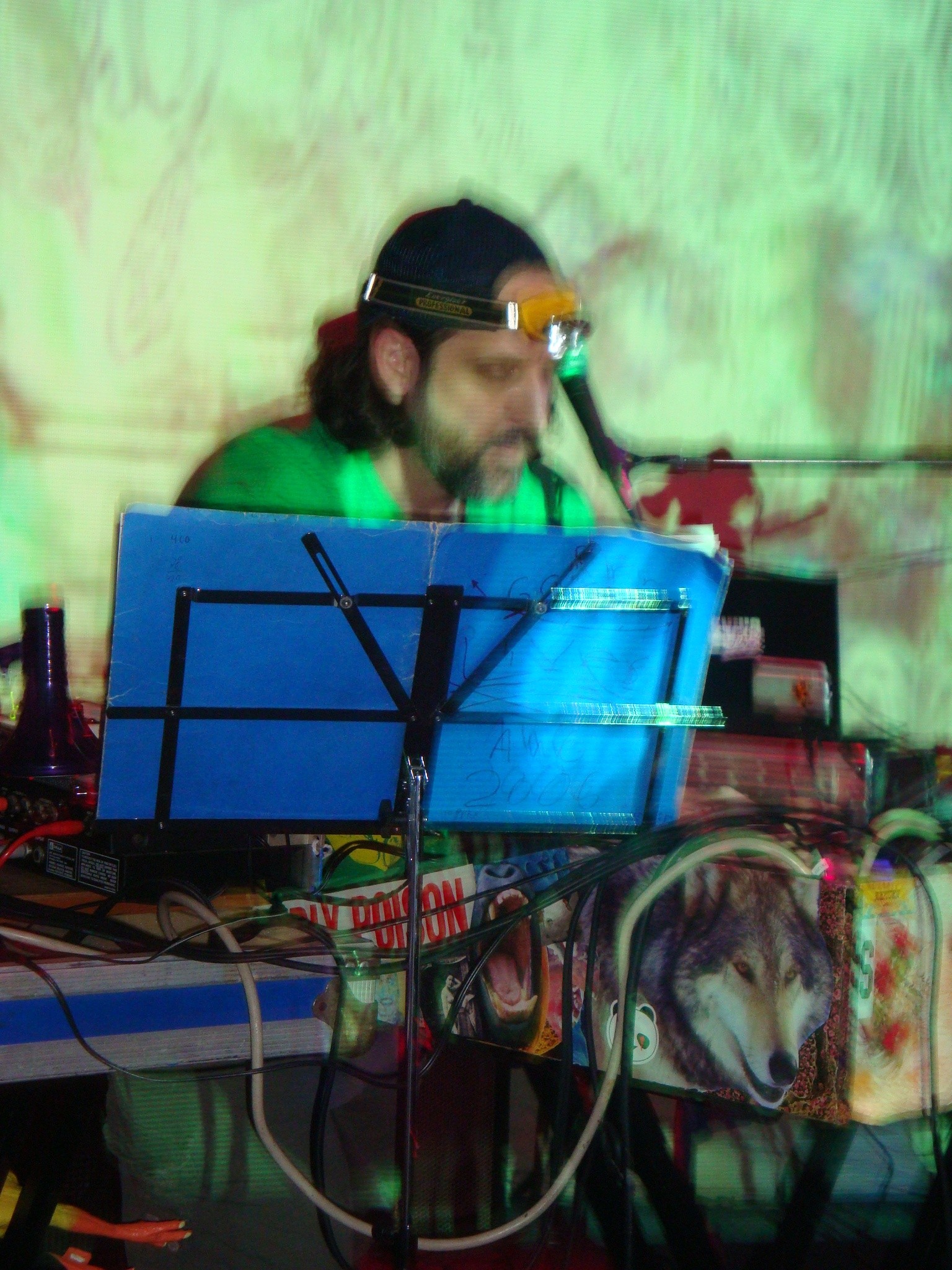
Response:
[181,200,593,883]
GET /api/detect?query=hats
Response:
[353,199,554,332]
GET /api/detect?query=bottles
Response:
[1,605,101,794]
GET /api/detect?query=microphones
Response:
[557,350,644,529]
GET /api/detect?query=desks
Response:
[0,911,330,1087]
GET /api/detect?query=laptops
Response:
[695,574,896,747]
